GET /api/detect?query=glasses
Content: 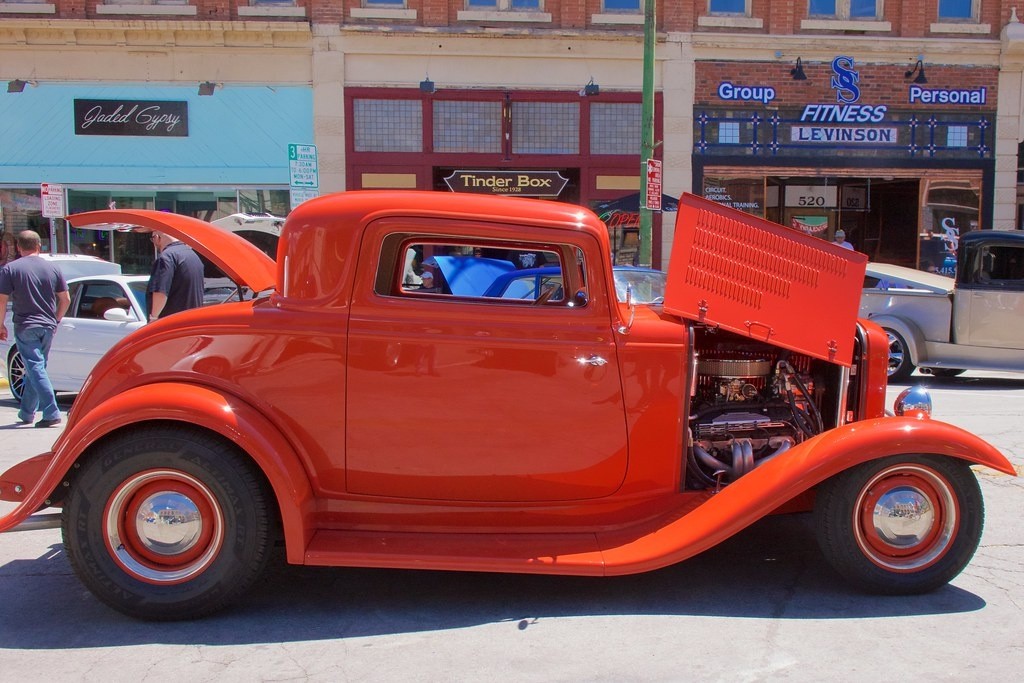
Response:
[149,235,161,242]
[475,253,482,256]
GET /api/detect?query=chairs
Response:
[90,298,120,318]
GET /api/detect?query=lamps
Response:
[198,81,216,95]
[906,58,927,83]
[582,78,601,97]
[791,58,807,80]
[7,80,37,92]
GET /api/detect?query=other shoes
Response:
[35,418,62,428]
[17,412,33,424]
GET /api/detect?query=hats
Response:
[835,230,846,238]
[420,271,433,280]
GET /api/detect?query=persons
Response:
[832,230,854,251]
[145,229,204,324]
[0,229,72,427]
[507,250,547,270]
[419,272,435,289]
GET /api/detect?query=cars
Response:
[856,228,1024,386]
[420,255,666,307]
[0,188,1021,624]
[0,211,286,404]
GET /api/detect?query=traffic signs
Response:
[286,142,319,189]
